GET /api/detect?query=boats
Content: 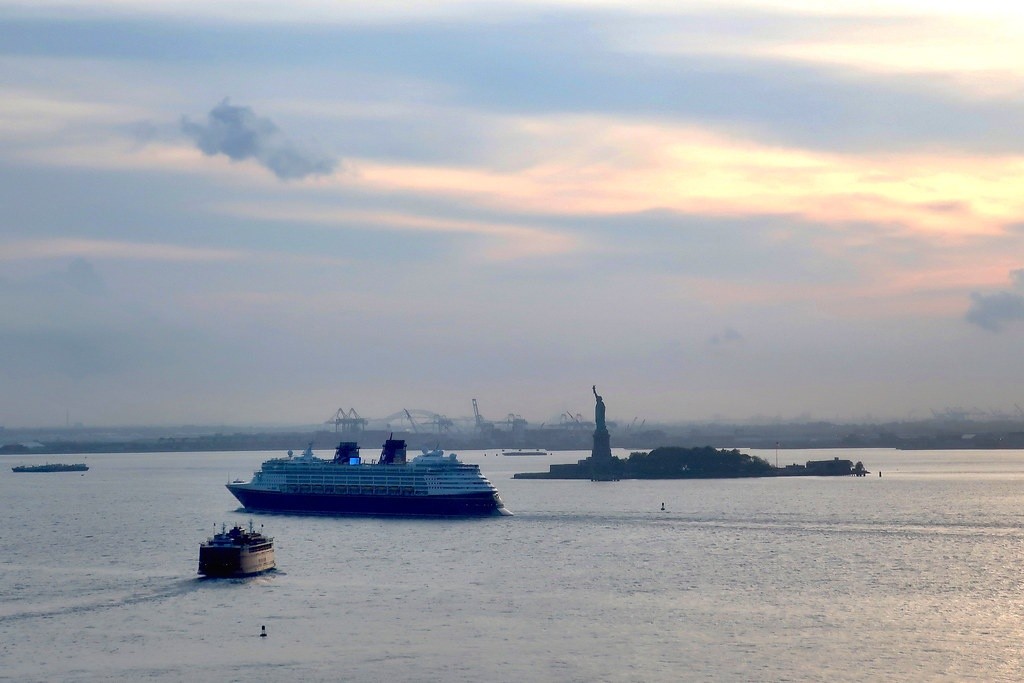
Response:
[325,485,334,491]
[501,451,548,456]
[196,513,277,578]
[349,486,360,492]
[312,486,323,491]
[375,486,387,492]
[11,457,90,473]
[388,487,399,492]
[401,487,414,493]
[335,486,346,492]
[361,486,374,493]
[288,485,298,491]
[300,485,311,491]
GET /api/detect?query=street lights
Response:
[775,442,779,468]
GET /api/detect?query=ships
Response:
[225,427,514,519]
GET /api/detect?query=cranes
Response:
[471,399,495,438]
[334,407,369,435]
[401,409,430,435]
